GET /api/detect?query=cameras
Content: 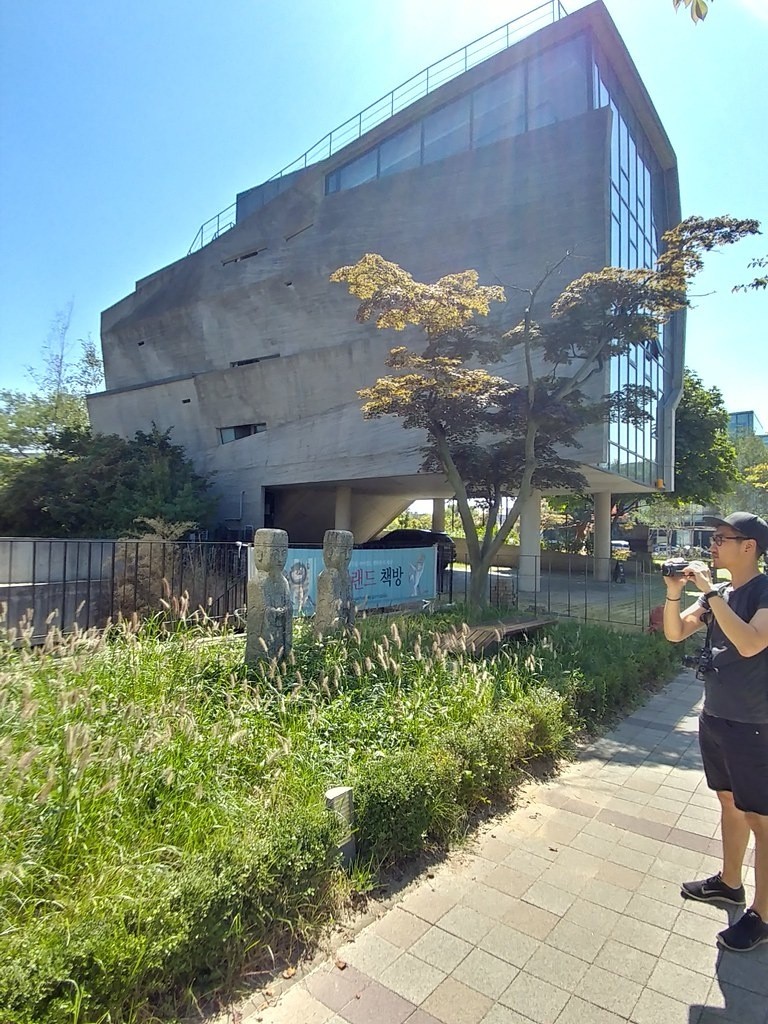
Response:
[662,561,695,577]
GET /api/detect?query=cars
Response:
[611,538,712,560]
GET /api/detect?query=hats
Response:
[702,512,768,550]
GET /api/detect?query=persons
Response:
[244,528,293,668]
[663,511,768,952]
[313,529,355,641]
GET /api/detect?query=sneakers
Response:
[681,871,746,905]
[716,908,768,951]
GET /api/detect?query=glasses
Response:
[710,535,750,546]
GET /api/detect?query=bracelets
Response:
[664,596,683,602]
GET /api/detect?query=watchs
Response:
[701,589,724,609]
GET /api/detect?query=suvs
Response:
[356,529,458,572]
